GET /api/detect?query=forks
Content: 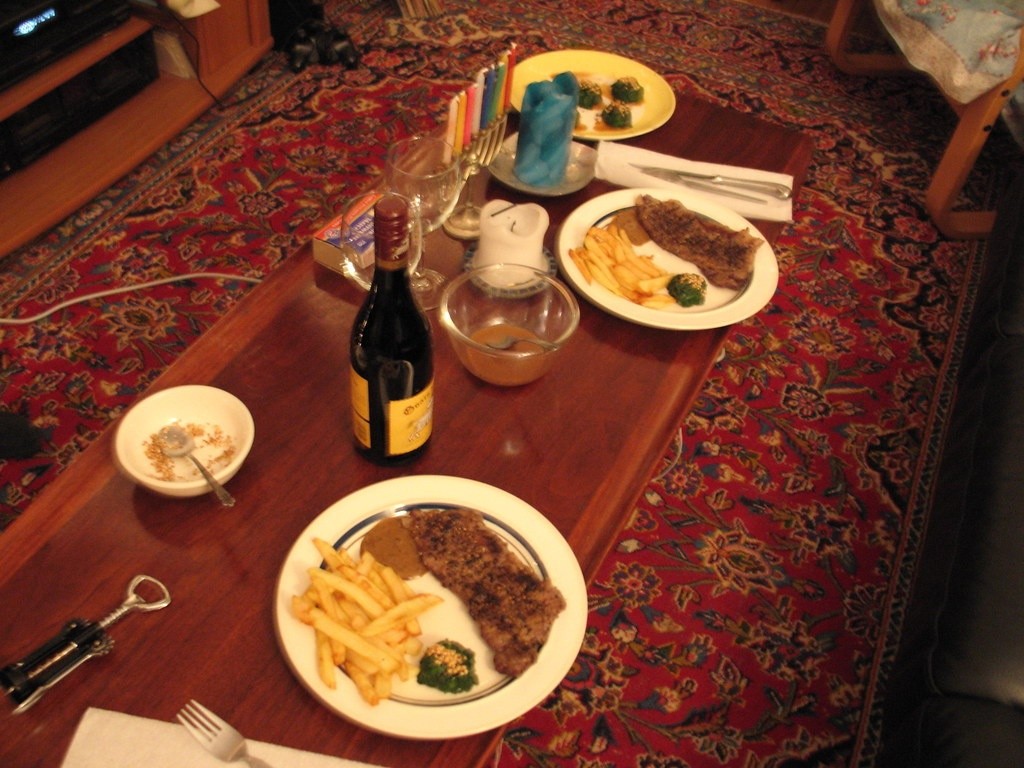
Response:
[641,167,767,205]
[175,699,274,768]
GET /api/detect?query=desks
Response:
[0,48,813,768]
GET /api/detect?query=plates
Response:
[510,49,676,140]
[272,474,588,740]
[554,187,779,331]
[487,132,597,196]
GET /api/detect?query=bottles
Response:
[349,194,433,468]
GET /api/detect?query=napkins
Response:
[60,706,383,768]
[591,140,798,226]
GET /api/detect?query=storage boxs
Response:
[310,186,382,275]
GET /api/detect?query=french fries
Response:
[570,224,679,307]
[290,537,445,705]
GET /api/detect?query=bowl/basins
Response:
[114,384,254,497]
[438,262,581,388]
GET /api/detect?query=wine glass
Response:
[382,136,459,311]
[340,186,422,292]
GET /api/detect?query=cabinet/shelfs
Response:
[0,0,271,268]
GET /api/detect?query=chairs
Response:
[822,1,1024,238]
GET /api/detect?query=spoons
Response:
[485,334,561,353]
[158,425,237,507]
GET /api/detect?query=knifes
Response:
[628,163,793,200]
